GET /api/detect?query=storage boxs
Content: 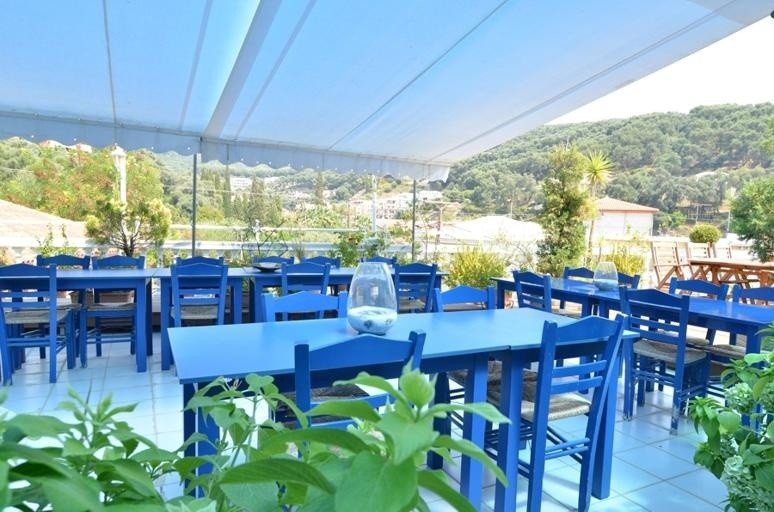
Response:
[650,242,774,304]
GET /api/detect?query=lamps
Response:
[260,291,379,425]
[278,329,426,512]
[486,313,628,512]
[433,285,536,481]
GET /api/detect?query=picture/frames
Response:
[166,307,640,512]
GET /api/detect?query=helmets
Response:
[253,262,281,273]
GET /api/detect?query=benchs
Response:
[650,242,774,304]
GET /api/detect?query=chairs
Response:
[433,285,536,481]
[260,291,379,425]
[278,329,426,512]
[0,253,449,386]
[510,265,773,434]
[486,313,628,512]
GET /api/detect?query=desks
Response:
[166,307,640,512]
